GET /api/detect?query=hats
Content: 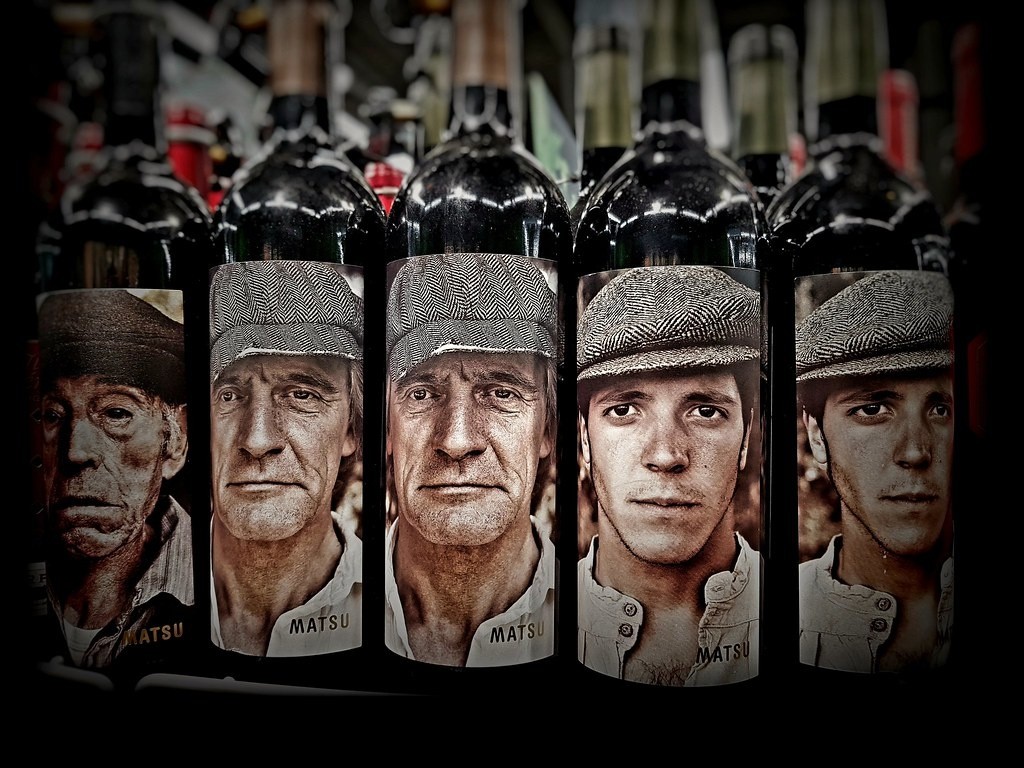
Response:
[39,290,184,405]
[386,253,558,383]
[210,260,365,384]
[796,271,954,388]
[577,265,760,385]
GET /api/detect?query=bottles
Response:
[0,0,970,698]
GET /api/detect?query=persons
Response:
[206,261,363,655]
[794,272,963,672]
[383,253,560,668]
[34,289,196,668]
[575,267,766,686]
